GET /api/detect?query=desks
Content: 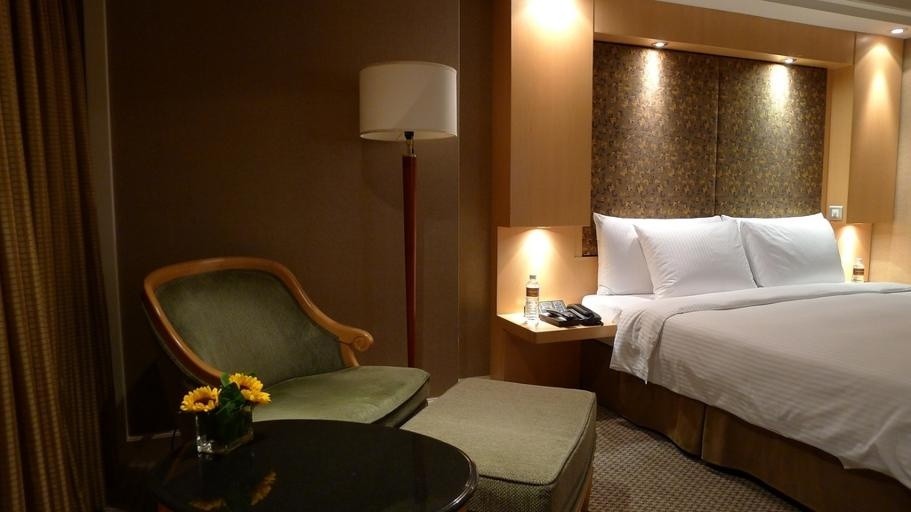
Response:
[102,418,477,508]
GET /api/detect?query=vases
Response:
[194,402,254,454]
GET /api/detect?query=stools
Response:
[399,376,599,512]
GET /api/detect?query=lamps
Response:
[354,58,458,368]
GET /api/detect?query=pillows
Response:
[592,208,845,301]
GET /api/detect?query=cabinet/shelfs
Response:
[491,0,593,226]
[846,32,904,226]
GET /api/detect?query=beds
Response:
[579,280,911,512]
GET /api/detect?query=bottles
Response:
[525,273,540,324]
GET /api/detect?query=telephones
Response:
[567,303,601,324]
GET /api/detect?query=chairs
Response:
[132,254,430,432]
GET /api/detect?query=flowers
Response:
[178,370,271,444]
